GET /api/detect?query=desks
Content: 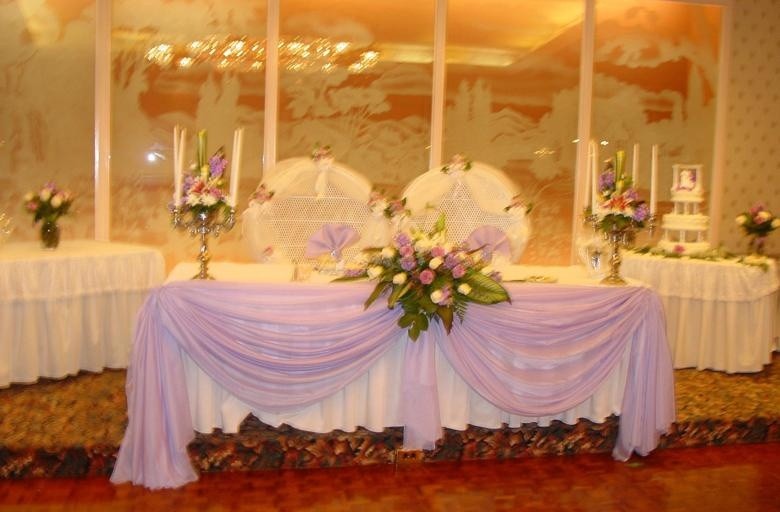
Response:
[1,241,165,383]
[107,248,670,492]
[585,241,780,372]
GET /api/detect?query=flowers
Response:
[169,128,230,218]
[735,204,780,235]
[22,180,75,221]
[583,160,658,232]
[334,216,511,341]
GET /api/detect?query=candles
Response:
[173,122,243,207]
[584,138,660,217]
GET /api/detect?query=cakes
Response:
[658,165,713,258]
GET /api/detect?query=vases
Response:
[40,222,60,249]
[750,237,772,263]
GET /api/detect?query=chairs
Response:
[247,155,532,274]
